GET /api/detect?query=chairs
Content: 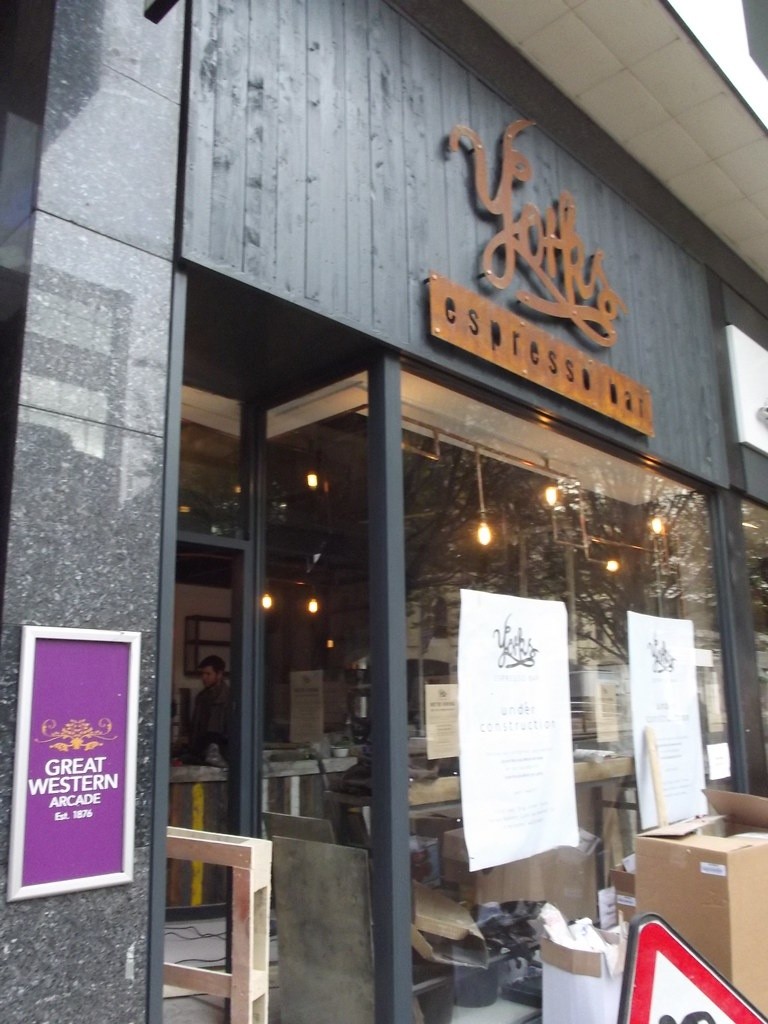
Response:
[262,809,336,847]
[271,835,424,1024]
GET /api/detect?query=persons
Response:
[191,654,237,762]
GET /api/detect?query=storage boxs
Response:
[407,784,767,1024]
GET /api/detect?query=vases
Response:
[415,944,504,1022]
